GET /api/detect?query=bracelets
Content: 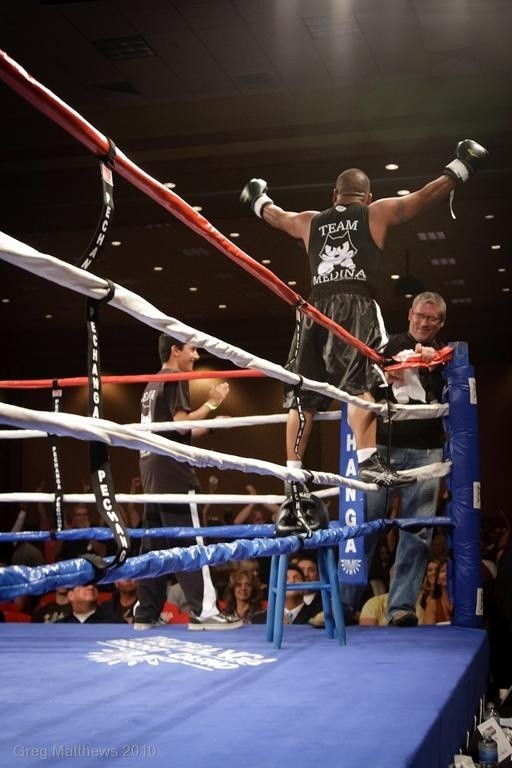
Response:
[204,399,217,411]
[209,428,215,436]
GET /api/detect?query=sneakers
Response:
[308,609,355,629]
[391,611,418,626]
[284,478,311,498]
[133,615,168,630]
[188,610,243,631]
[356,451,418,488]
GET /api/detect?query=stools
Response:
[262,532,346,647]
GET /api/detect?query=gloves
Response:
[240,177,274,219]
[444,138,488,185]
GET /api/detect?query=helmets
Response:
[273,492,329,538]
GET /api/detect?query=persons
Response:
[241,137,489,499]
[309,291,448,627]
[129,332,244,630]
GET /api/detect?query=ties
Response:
[286,612,293,624]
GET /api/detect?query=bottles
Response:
[477,730,498,768]
[483,702,500,728]
